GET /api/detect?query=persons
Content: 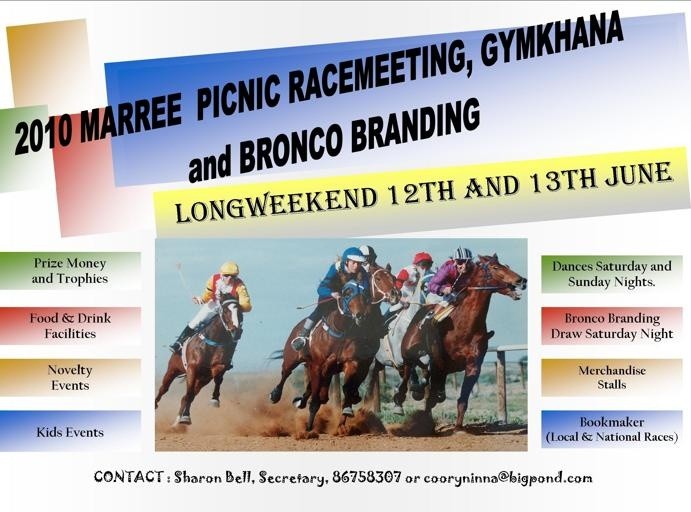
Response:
[426,247,497,340]
[168,261,253,408]
[382,250,435,322]
[292,246,370,353]
[361,247,378,272]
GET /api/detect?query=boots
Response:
[167,325,195,354]
[291,327,310,352]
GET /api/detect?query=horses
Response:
[155,289,244,432]
[269,251,528,437]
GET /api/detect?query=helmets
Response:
[453,248,473,260]
[342,245,377,262]
[220,262,239,275]
[413,252,433,265]
